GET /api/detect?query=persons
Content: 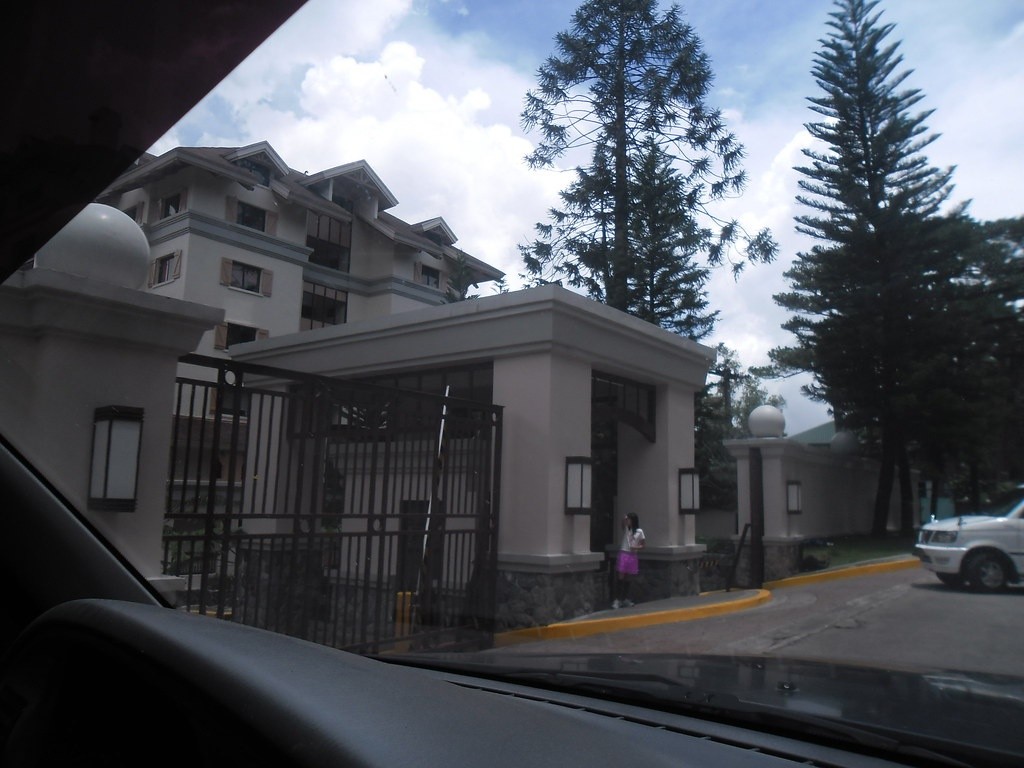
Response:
[611,510,646,609]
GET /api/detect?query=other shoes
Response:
[622,599,635,607]
[611,598,624,609]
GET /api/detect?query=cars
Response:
[911,491,1024,597]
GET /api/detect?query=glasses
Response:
[624,518,629,520]
